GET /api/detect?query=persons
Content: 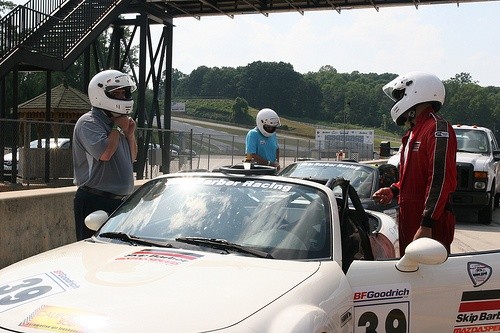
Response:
[245,108,281,171]
[374,72,458,259]
[72,69,137,242]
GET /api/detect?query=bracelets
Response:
[267,160,270,165]
[112,124,124,135]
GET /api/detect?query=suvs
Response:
[386,124,500,225]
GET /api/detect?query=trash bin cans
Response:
[380,141,391,157]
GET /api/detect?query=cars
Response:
[255,158,400,225]
[4,138,71,173]
[0,161,500,333]
[143,142,196,161]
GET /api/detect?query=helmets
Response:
[390,72,445,126]
[88,70,134,114]
[256,108,281,137]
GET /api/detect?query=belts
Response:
[79,186,128,200]
